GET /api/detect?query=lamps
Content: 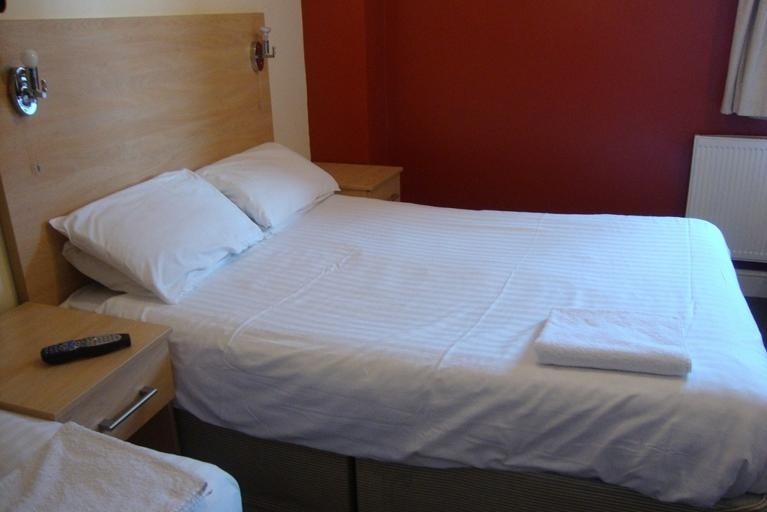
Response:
[8,49,48,117]
[249,26,275,73]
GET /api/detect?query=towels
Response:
[532,307,692,376]
[0,420,212,511]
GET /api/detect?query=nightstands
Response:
[0,301,182,457]
[312,160,404,203]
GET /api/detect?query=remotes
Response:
[40,333,130,364]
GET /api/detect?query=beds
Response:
[58,194,767,510]
